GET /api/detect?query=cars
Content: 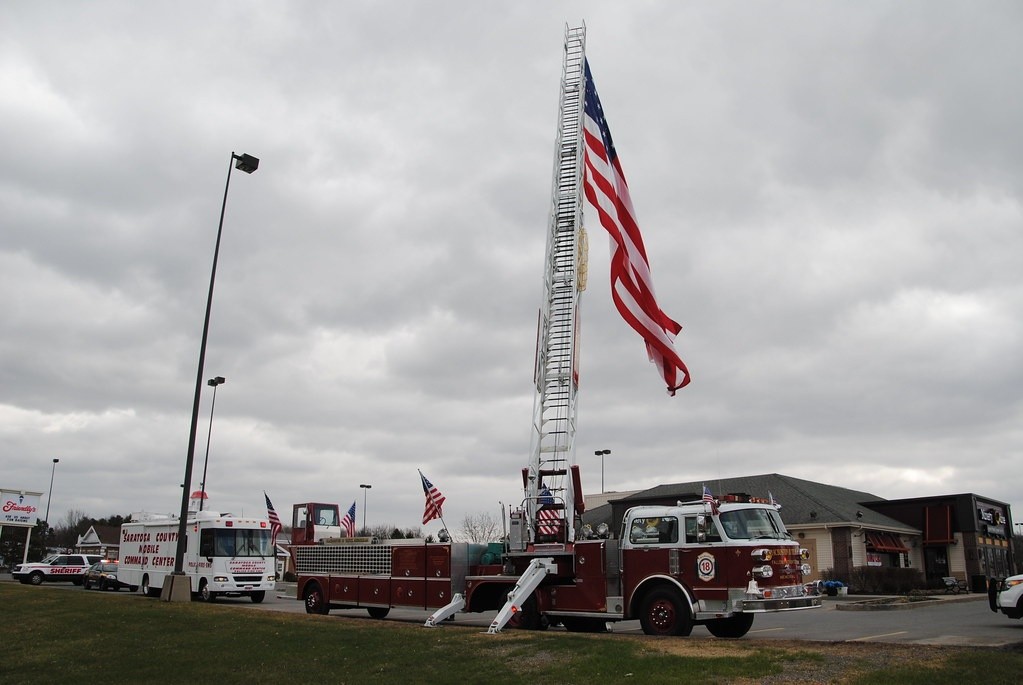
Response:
[83,560,140,593]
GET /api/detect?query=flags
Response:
[537,484,559,534]
[583,59,691,397]
[704,487,721,515]
[265,494,282,547]
[422,474,446,525]
[340,502,356,538]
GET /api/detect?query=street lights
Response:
[360,485,371,536]
[171,151,261,575]
[199,376,225,512]
[595,450,611,493]
[1015,523,1023,536]
[39,459,60,560]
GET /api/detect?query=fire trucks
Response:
[286,19,822,637]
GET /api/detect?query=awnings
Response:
[867,532,909,554]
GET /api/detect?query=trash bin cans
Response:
[836,587,848,598]
[970,574,987,593]
[825,586,838,597]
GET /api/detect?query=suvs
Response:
[988,573,1023,619]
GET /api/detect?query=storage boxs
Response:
[837,586,848,595]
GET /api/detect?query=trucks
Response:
[117,510,275,602]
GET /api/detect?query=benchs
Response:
[941,577,969,595]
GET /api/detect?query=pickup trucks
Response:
[12,553,106,586]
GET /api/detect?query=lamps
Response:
[810,510,817,520]
[856,511,864,520]
